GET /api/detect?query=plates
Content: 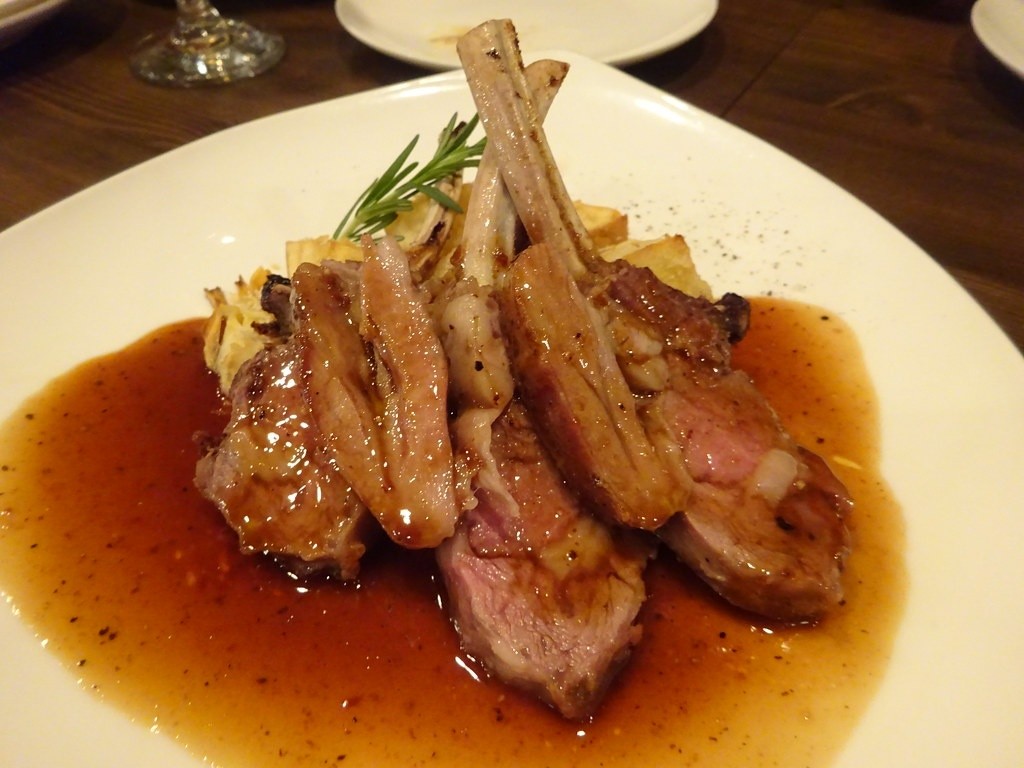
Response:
[1,0,66,35]
[970,1,1023,78]
[333,1,721,71]
[0,49,1024,767]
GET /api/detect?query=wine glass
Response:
[128,1,287,87]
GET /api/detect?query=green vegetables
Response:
[331,108,491,244]
